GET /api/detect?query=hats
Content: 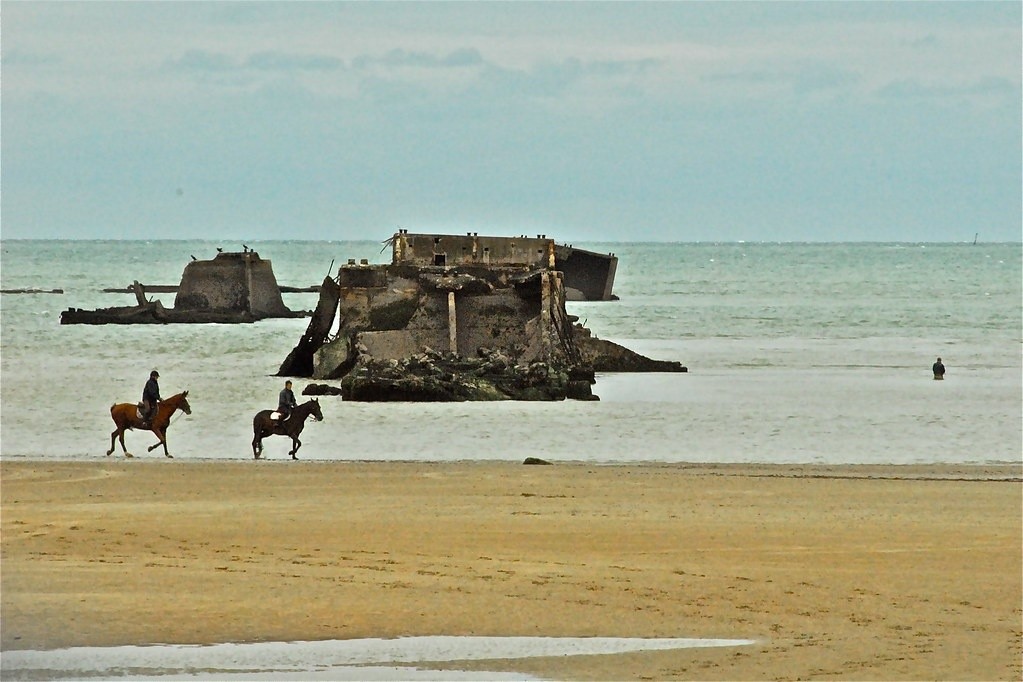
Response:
[150,370,160,378]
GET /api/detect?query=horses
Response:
[251,397,324,460]
[106,390,192,458]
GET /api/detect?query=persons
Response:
[142,371,164,425]
[933,357,945,379]
[276,381,297,424]
